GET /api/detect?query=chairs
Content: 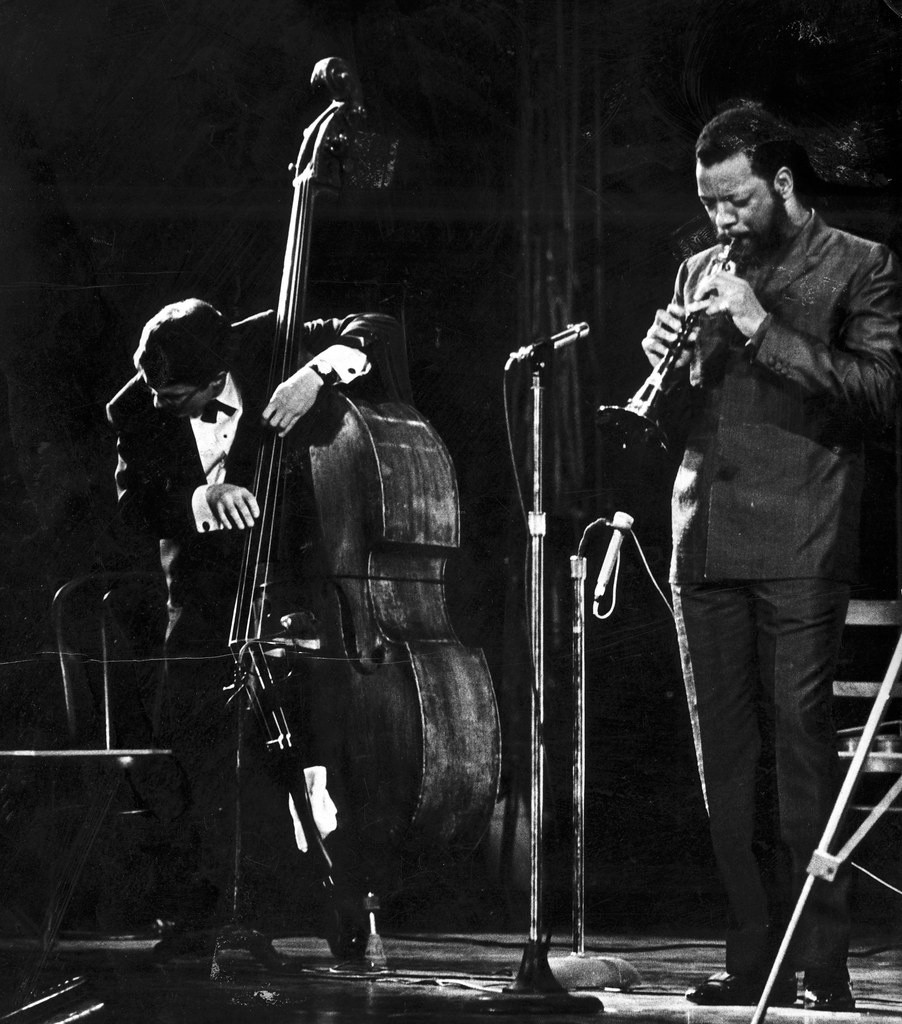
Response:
[830,600,902,901]
[0,573,182,967]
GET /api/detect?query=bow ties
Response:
[201,400,235,423]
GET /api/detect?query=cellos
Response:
[177,52,507,970]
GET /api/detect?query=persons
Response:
[642,103,901,1011]
[107,298,401,984]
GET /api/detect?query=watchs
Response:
[306,358,340,386]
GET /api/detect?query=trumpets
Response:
[590,229,747,470]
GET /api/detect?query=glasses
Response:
[156,386,207,413]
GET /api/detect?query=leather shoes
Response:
[685,971,799,1008]
[803,985,858,1013]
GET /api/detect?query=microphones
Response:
[591,509,634,601]
[506,322,590,371]
